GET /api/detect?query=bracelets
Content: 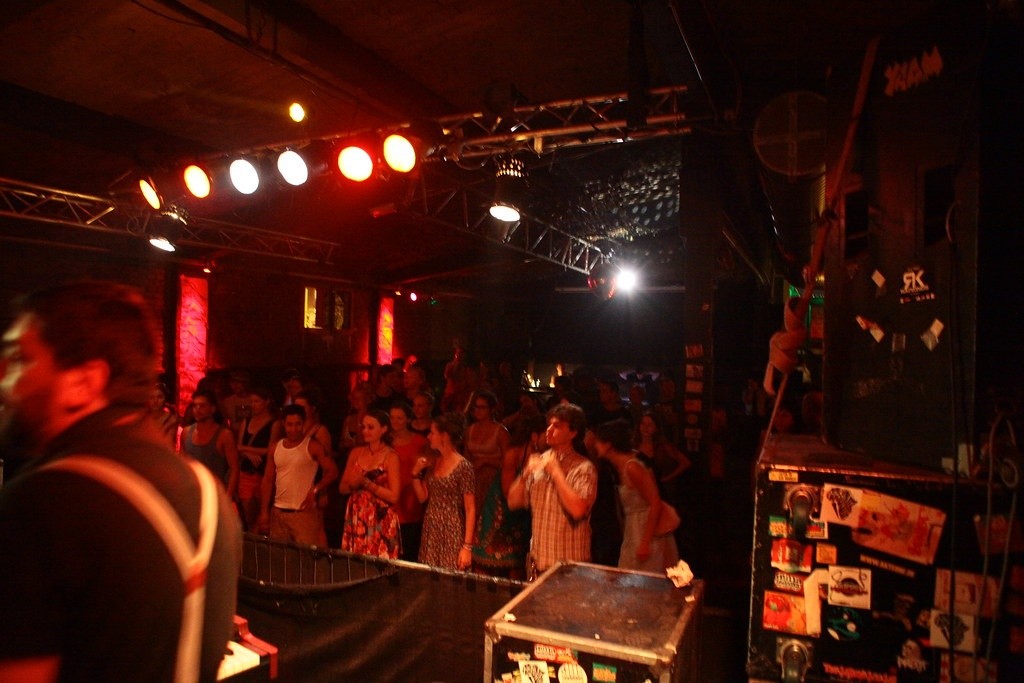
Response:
[465,542,473,547]
[462,544,473,552]
[363,477,372,488]
[311,486,319,494]
[412,475,421,480]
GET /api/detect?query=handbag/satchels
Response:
[653,500,680,536]
[507,513,532,545]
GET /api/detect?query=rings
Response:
[423,458,426,462]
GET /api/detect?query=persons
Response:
[150,335,824,576]
[0,278,242,683]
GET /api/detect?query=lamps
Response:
[139,121,445,251]
[489,155,527,222]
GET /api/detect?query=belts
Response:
[282,508,296,512]
